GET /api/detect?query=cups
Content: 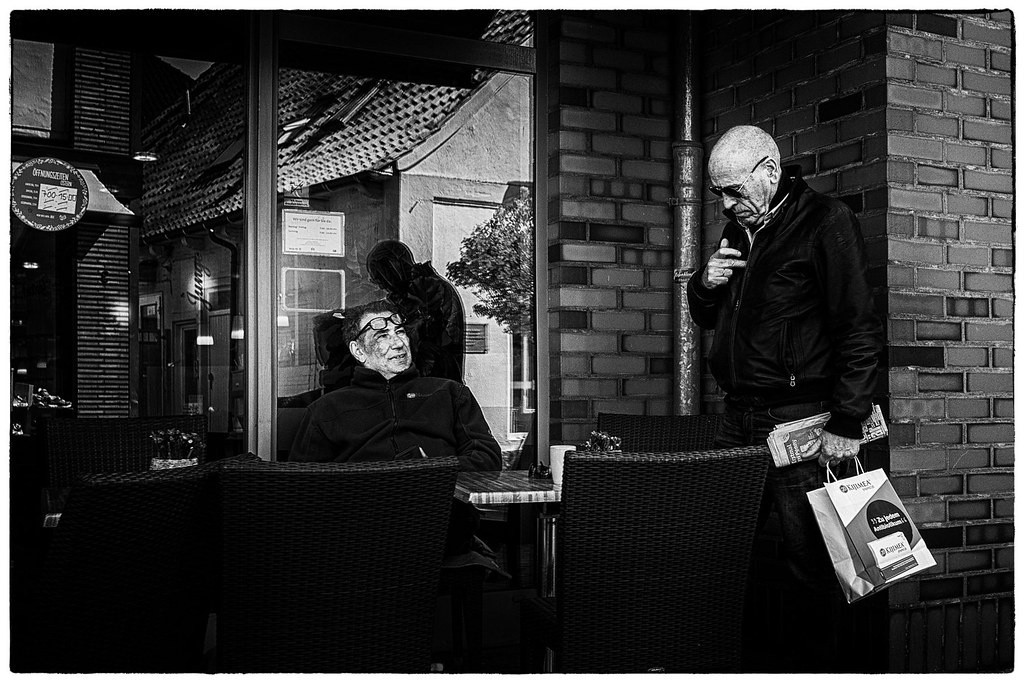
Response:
[549,445,576,485]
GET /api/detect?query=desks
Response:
[454,470,564,599]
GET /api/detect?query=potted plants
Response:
[147,428,206,471]
[578,431,622,454]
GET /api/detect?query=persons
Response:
[686,126,878,675]
[342,240,463,385]
[288,298,503,675]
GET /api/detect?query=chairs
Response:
[10,412,771,673]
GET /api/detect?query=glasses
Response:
[709,154,769,199]
[353,312,407,340]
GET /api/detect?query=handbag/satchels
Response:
[805,455,938,604]
